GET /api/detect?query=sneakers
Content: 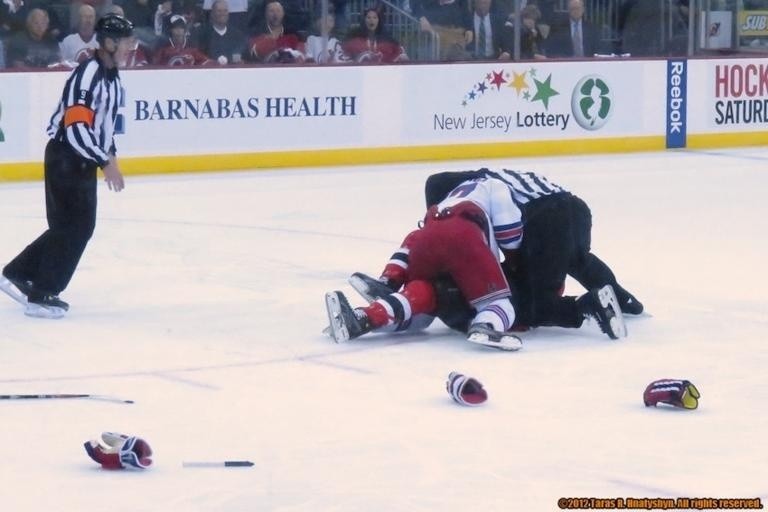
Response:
[334,290,371,340]
[621,295,643,315]
[352,272,398,300]
[575,289,618,340]
[27,295,69,310]
[467,323,523,344]
[2,267,32,296]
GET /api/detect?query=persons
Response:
[334,168,644,350]
[2,12,134,309]
[0,1,602,70]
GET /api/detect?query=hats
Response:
[167,13,186,29]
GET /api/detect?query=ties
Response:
[572,21,582,56]
[476,18,487,57]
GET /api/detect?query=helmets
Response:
[95,12,137,68]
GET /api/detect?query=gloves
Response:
[446,372,489,406]
[84,432,153,470]
[644,380,700,410]
